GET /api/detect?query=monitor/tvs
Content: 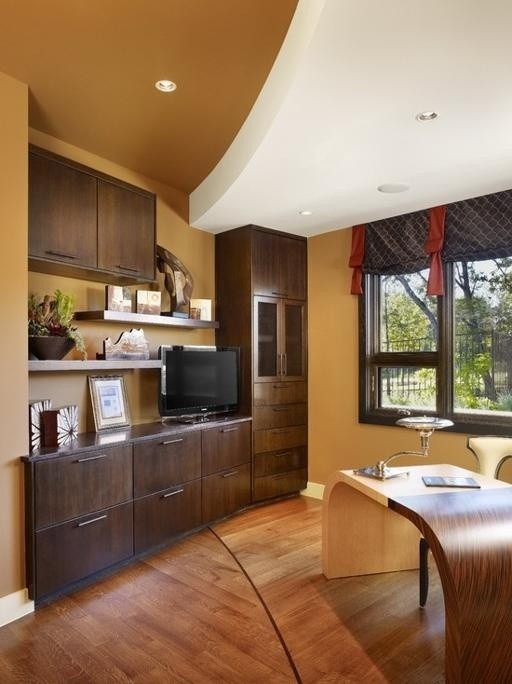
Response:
[158,344,242,424]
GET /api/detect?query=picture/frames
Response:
[88,374,131,433]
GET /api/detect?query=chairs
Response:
[420,435,511,606]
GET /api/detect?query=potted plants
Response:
[28,290,88,360]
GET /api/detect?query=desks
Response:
[322,463,511,684]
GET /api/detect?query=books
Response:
[422,476,481,488]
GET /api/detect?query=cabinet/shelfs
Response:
[201,422,252,525]
[28,309,220,370]
[28,141,156,286]
[33,443,134,598]
[215,223,309,508]
[133,431,201,556]
